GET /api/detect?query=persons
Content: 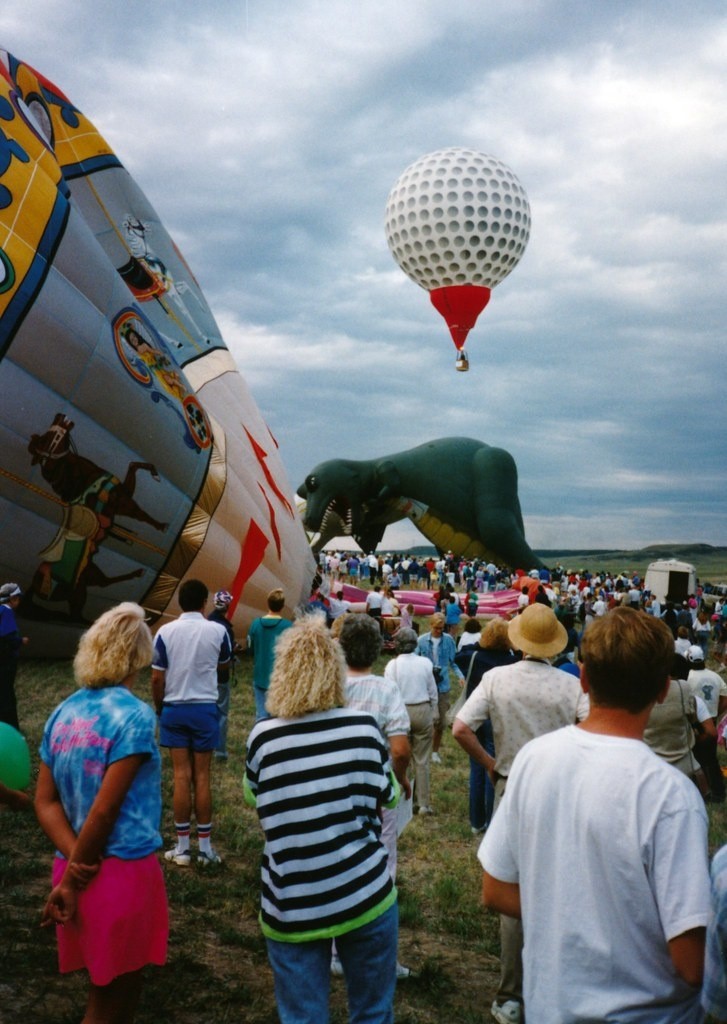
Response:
[151,580,233,868]
[0,550,727,1024]
[475,608,712,1024]
[245,589,293,721]
[244,622,402,1024]
[35,602,170,1024]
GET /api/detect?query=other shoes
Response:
[471,823,487,834]
[164,848,191,867]
[330,956,344,977]
[432,752,441,764]
[396,962,409,978]
[197,851,222,867]
[418,807,434,815]
[492,1000,522,1024]
[716,666,726,672]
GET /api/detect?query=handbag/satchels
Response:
[445,683,468,728]
[693,769,711,799]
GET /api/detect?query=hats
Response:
[508,603,568,658]
[212,590,234,610]
[0,583,23,602]
[688,645,705,663]
[710,614,719,621]
[587,593,593,597]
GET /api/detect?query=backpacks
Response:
[719,624,727,643]
